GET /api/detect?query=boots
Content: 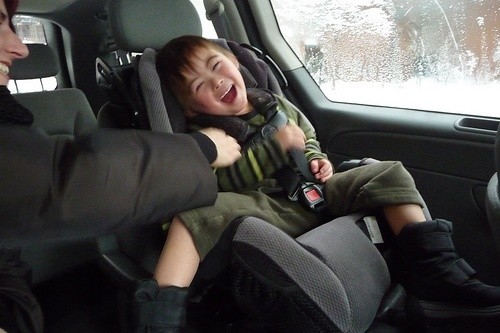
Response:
[127,279,190,333]
[395,220,500,318]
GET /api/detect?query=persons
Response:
[0,0,240,333]
[129,35,500,333]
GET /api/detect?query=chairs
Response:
[93,1,437,333]
[5,42,102,144]
